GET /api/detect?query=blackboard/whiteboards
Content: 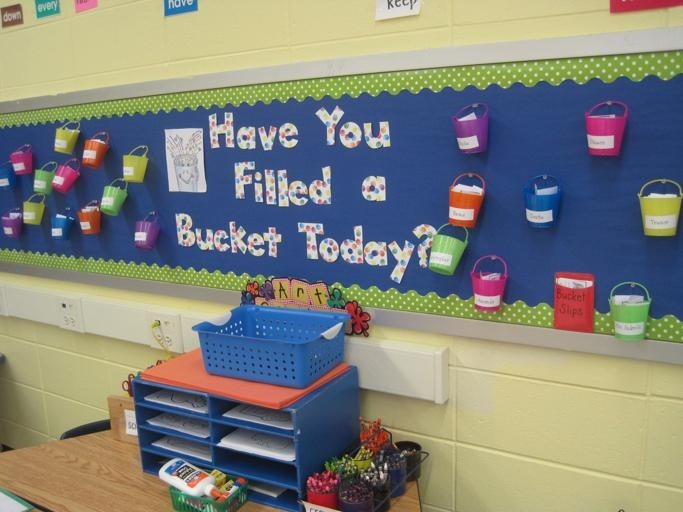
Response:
[0,26,682,366]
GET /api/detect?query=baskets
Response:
[168,470,249,512]
[191,303,351,390]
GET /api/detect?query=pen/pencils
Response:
[306,416,416,502]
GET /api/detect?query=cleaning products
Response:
[159,457,226,501]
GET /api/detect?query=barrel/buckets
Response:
[470,255,509,310]
[50,208,75,240]
[134,211,161,250]
[447,172,485,226]
[52,157,82,193]
[99,178,129,217]
[80,131,110,167]
[8,144,32,174]
[607,281,652,341]
[450,102,489,153]
[54,121,81,155]
[521,174,560,228]
[636,175,683,237]
[428,223,469,275]
[34,161,58,196]
[0,160,16,192]
[20,193,46,225]
[122,146,150,182]
[2,209,23,239]
[77,200,101,235]
[585,100,627,155]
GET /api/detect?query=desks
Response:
[0,432,423,512]
